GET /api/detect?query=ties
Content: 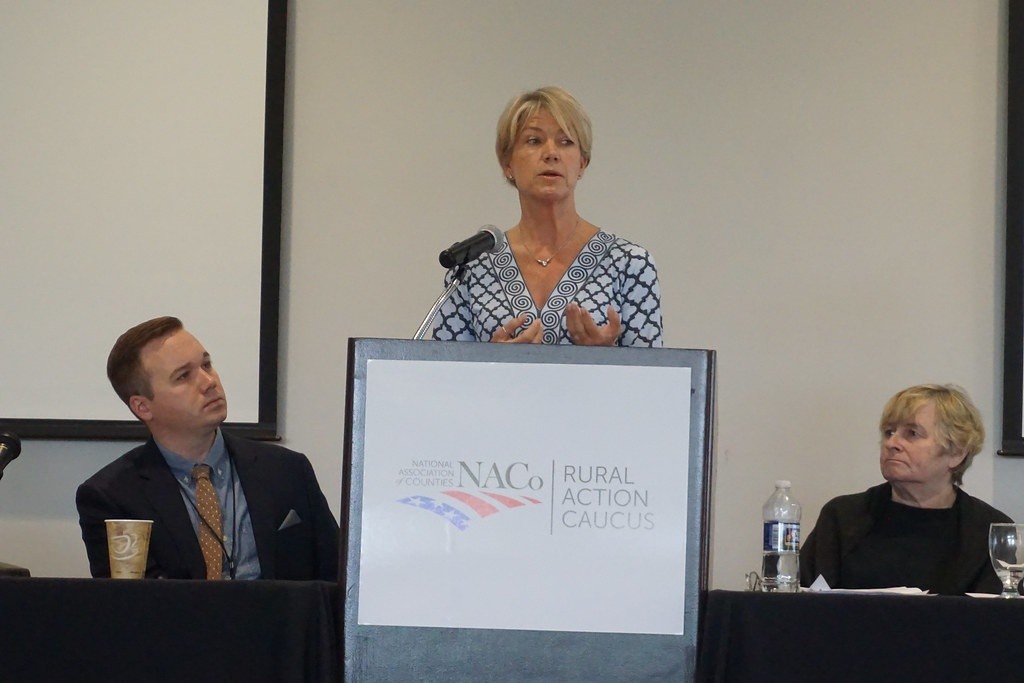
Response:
[191,463,223,581]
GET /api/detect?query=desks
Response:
[0,577,1024,683]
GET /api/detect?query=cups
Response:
[103,519,154,579]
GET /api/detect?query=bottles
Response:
[762,479,803,596]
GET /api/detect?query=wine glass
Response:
[988,522,1024,600]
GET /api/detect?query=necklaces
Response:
[171,452,237,581]
[519,216,580,266]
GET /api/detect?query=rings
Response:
[502,326,511,337]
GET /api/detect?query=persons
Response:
[797,384,1016,597]
[76,316,341,582]
[432,86,663,347]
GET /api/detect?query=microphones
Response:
[0,431,21,480]
[439,225,504,268]
